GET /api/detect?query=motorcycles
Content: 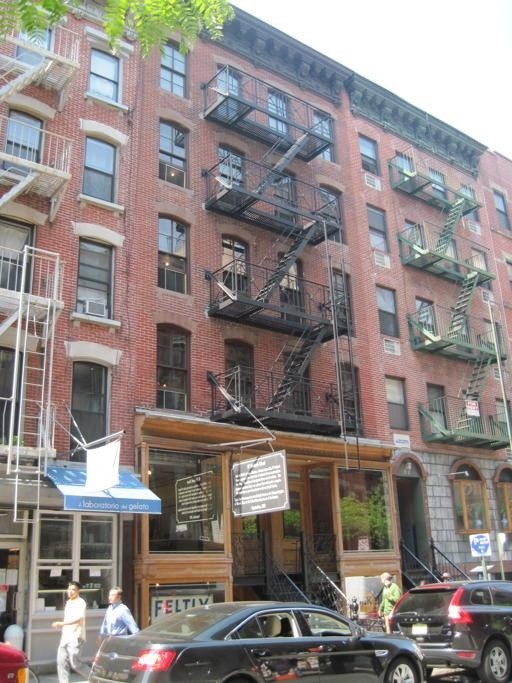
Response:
[249,647,275,682]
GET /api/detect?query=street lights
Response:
[495,530,507,581]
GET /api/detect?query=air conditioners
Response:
[83,301,105,317]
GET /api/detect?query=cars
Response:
[38,540,113,589]
[0,640,30,683]
[85,599,430,683]
[271,641,382,681]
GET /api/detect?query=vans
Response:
[387,578,512,683]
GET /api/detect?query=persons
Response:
[48,579,94,683]
[97,585,139,642]
[275,617,294,636]
[376,571,403,635]
